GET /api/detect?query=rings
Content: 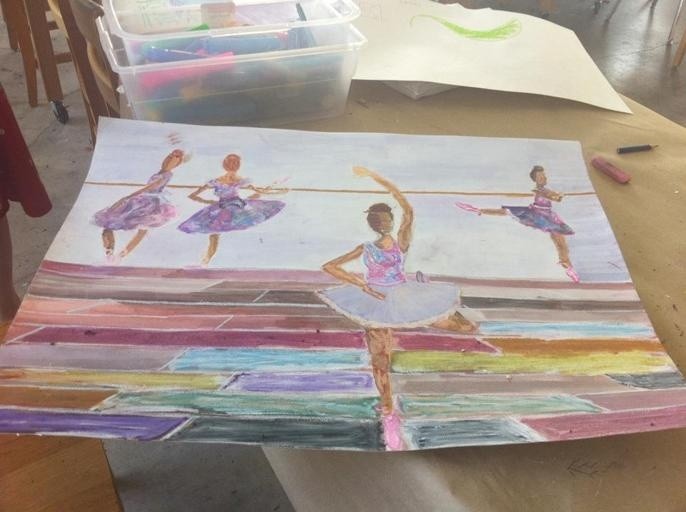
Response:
[129,3,333,125]
[617,144,660,153]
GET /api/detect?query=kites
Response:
[592,157,631,184]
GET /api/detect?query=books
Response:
[96,3,367,126]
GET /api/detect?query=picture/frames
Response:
[260,80,685,512]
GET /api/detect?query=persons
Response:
[453,165,579,283]
[313,162,484,452]
[91,148,192,267]
[177,152,294,271]
